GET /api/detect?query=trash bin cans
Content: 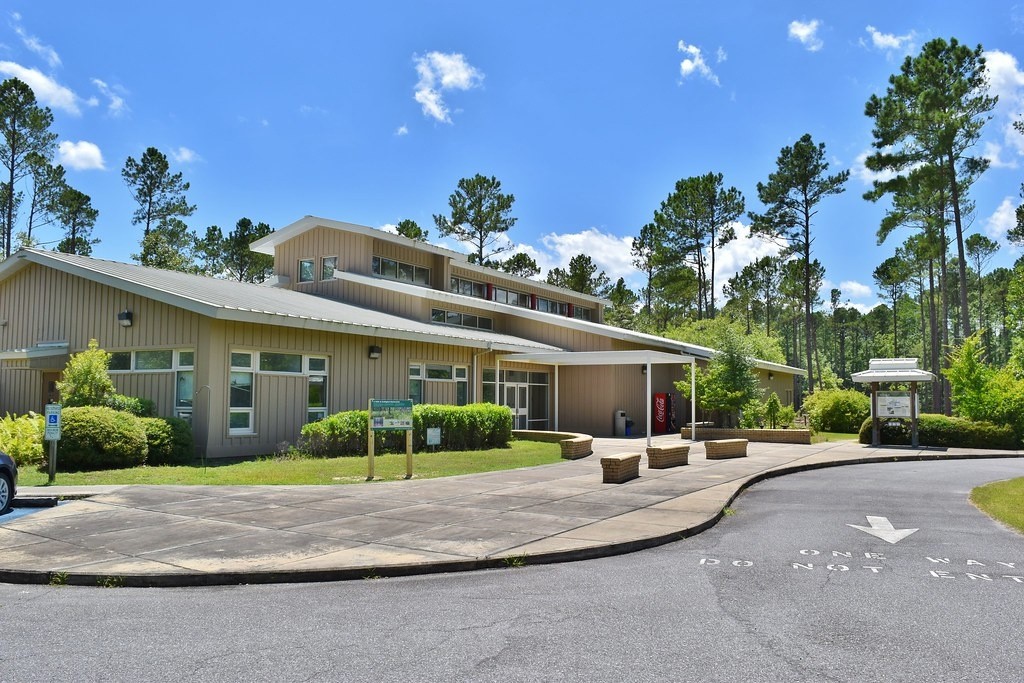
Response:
[615,410,626,436]
[626,421,632,436]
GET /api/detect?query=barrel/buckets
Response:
[626,420,633,436]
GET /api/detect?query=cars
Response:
[0,451,19,514]
